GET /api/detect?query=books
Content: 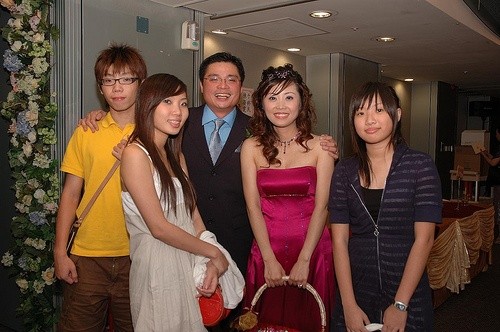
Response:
[472,142,486,155]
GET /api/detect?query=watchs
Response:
[391,299,408,312]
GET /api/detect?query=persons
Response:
[78,51,339,332]
[119,73,229,332]
[480,127,500,246]
[239,63,335,332]
[53,42,148,332]
[329,81,442,332]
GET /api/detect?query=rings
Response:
[298,284,302,287]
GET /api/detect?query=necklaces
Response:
[275,130,300,154]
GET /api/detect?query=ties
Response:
[208,119,226,165]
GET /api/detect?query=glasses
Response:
[203,76,240,85]
[101,77,139,86]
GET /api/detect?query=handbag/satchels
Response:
[230,275,326,332]
[65,215,80,256]
[198,290,230,327]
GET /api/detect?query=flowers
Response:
[0,0,61,332]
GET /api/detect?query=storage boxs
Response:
[453,145,486,176]
[461,130,490,153]
